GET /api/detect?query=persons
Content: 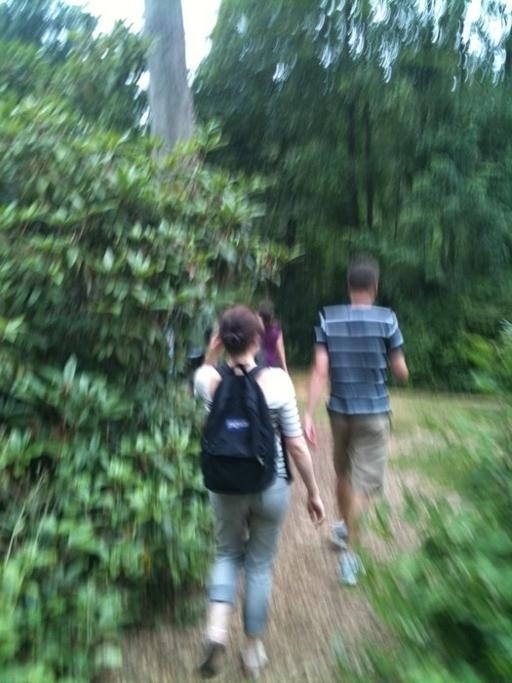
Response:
[192,305,326,682]
[257,298,288,374]
[301,261,410,587]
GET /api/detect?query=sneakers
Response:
[327,520,362,586]
[195,630,270,682]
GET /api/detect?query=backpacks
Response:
[197,361,279,493]
